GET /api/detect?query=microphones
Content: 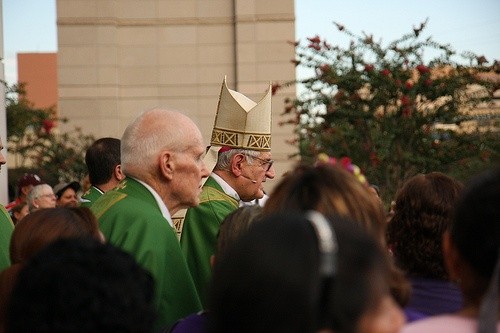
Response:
[224,169,257,183]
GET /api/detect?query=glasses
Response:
[246,154,274,172]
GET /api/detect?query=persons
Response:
[0,108,500,333]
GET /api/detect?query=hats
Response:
[54,181,81,195]
[18,174,46,187]
[210,75,273,153]
